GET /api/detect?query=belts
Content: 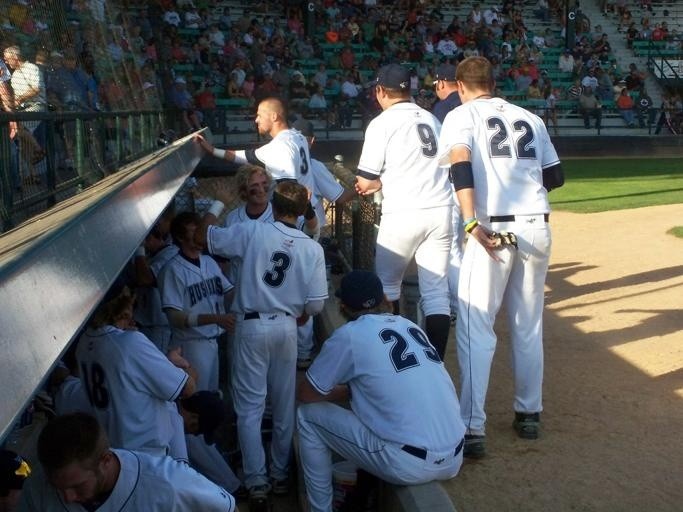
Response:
[489,213,550,223]
[403,438,464,462]
[245,312,292,320]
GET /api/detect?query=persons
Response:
[1,1,683,198]
[0,57,564,512]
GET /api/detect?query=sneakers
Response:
[513,412,539,440]
[463,439,487,459]
[234,478,291,501]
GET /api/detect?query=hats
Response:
[191,390,224,437]
[431,64,456,82]
[364,63,411,91]
[334,270,384,310]
[175,78,187,84]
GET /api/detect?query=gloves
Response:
[490,230,519,249]
